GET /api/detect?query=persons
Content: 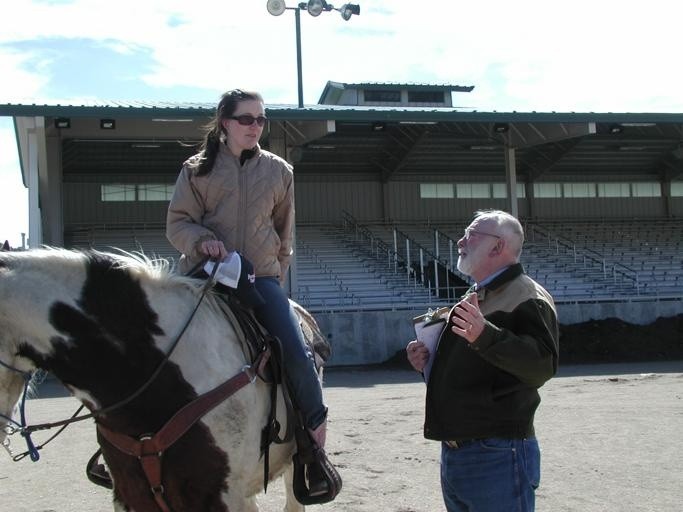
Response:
[404,208,561,512]
[87,88,332,499]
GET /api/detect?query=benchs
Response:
[63,219,682,310]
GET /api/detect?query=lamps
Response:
[494,124,508,132]
[54,118,68,128]
[372,122,384,133]
[101,119,115,129]
[611,124,621,133]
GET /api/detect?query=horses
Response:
[0,243,324,512]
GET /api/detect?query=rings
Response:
[467,322,473,332]
[207,247,214,251]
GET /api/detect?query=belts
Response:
[444,434,495,449]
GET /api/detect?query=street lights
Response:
[266,0,360,107]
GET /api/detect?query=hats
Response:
[204,249,267,306]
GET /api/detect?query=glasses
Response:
[464,229,500,240]
[227,115,267,127]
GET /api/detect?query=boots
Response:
[299,413,330,497]
